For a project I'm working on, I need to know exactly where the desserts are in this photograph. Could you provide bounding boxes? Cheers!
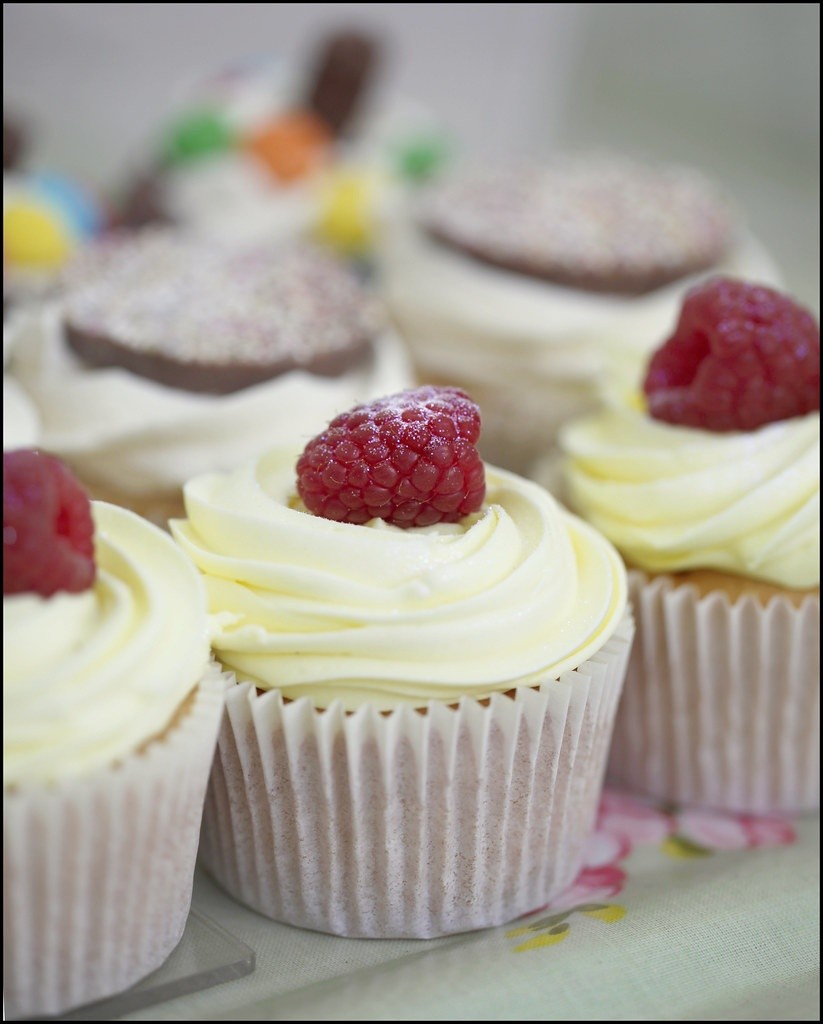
[2,30,821,1024]
